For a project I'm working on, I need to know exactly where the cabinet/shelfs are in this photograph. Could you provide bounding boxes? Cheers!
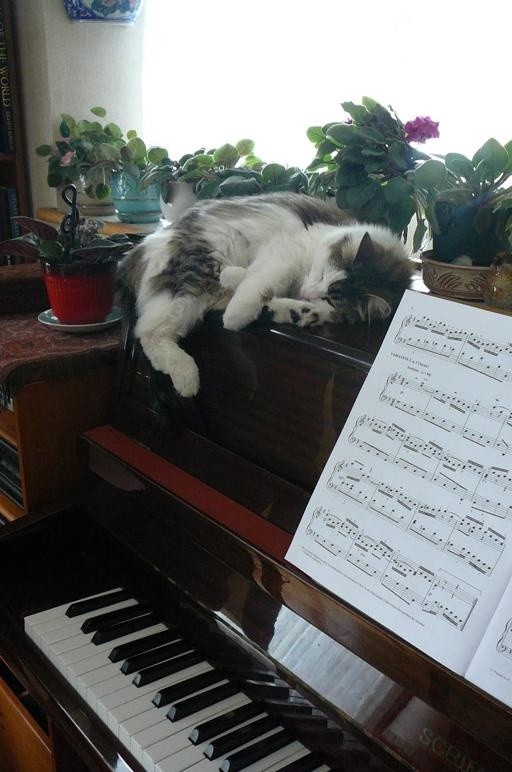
[0,261,119,526]
[0,0,33,265]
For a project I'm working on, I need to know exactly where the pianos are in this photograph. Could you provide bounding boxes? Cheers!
[0,280,512,772]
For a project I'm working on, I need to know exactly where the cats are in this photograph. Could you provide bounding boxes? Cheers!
[109,190,416,451]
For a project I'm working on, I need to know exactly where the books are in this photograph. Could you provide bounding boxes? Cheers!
[283,287,512,714]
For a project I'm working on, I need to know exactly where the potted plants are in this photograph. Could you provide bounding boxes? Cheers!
[0,96,512,336]
